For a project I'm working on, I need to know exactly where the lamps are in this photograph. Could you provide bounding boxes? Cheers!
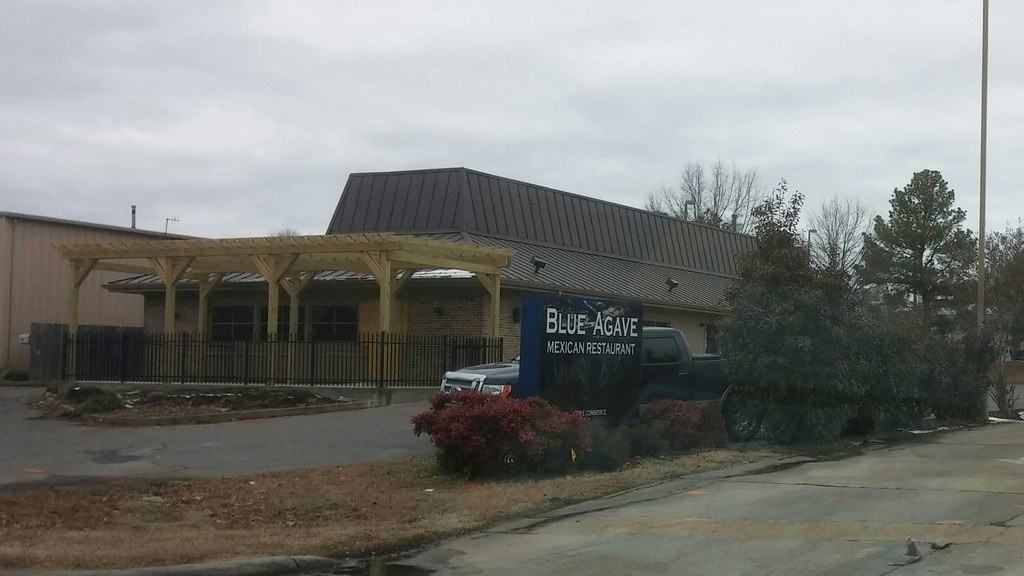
[531,255,546,274]
[174,312,180,318]
[665,277,679,292]
[433,301,440,313]
[699,323,705,327]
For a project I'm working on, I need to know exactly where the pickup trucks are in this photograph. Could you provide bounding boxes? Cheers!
[441,325,766,442]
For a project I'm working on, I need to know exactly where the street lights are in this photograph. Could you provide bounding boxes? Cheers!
[684,200,695,219]
[809,229,816,254]
